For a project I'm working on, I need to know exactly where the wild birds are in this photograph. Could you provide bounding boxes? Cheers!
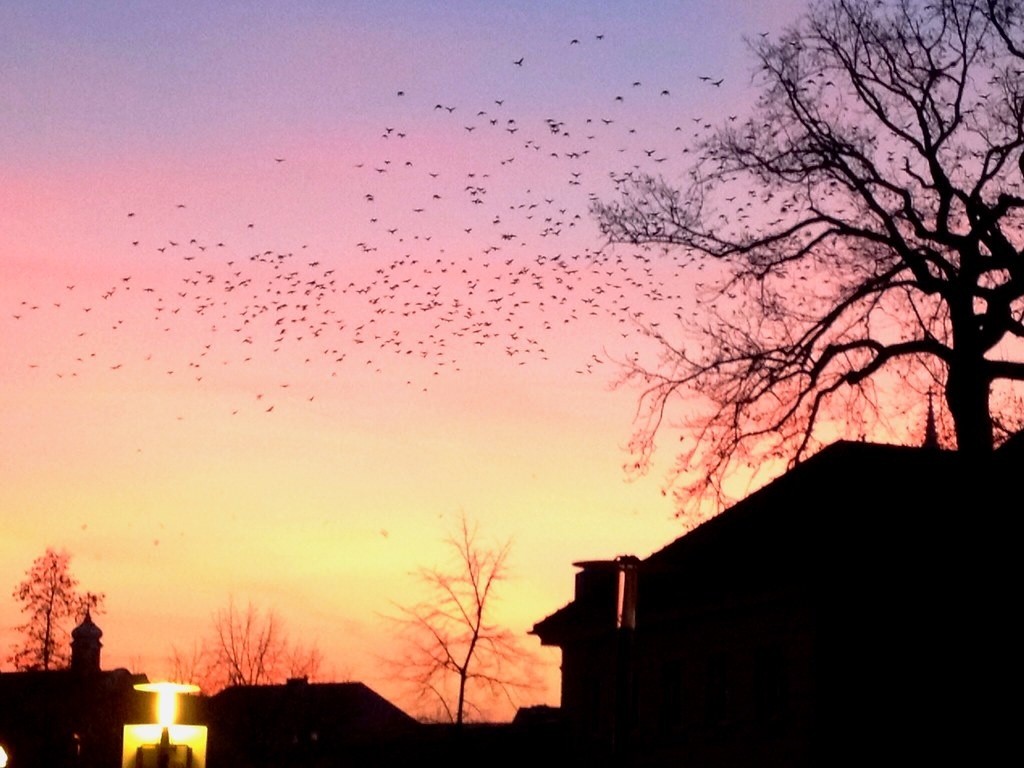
[11,4,1023,423]
